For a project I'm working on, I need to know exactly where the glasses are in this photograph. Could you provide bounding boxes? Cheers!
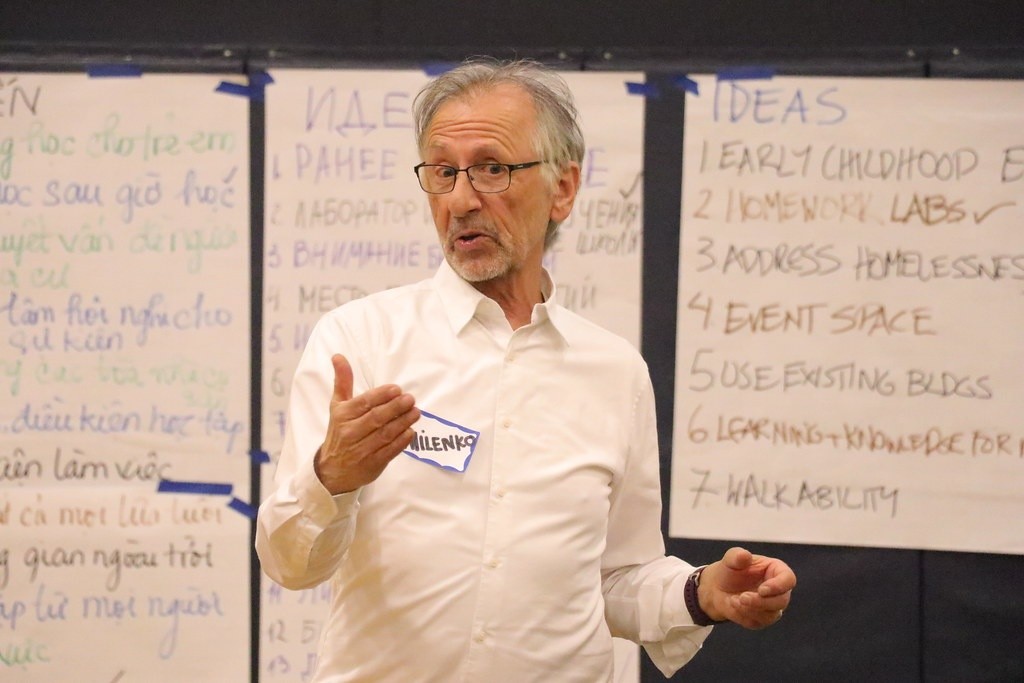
[414,160,548,194]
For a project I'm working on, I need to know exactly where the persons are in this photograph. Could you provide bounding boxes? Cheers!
[256,62,796,683]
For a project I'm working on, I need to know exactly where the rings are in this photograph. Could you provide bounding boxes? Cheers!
[779,609,783,620]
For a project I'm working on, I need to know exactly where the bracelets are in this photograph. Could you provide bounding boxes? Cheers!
[683,566,721,626]
